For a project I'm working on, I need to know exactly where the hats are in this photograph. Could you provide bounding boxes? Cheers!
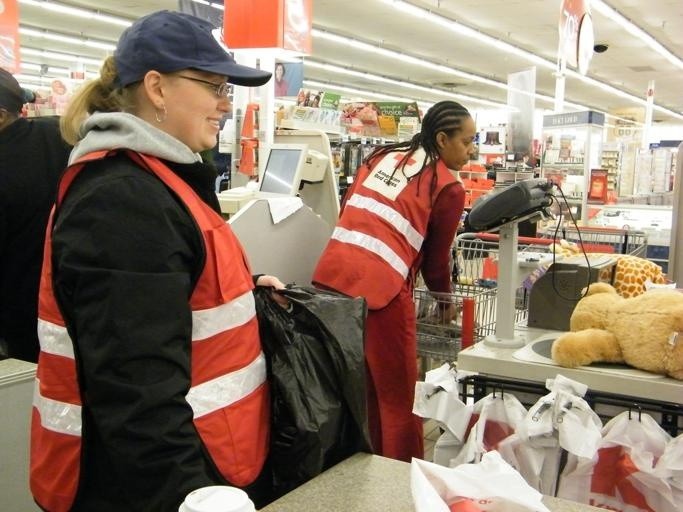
[114,10,272,88]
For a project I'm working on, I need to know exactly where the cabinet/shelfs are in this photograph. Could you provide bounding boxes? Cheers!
[447,110,683,275]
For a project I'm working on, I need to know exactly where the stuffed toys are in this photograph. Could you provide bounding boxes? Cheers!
[550,281,683,383]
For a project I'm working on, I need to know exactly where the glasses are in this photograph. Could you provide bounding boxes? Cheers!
[124,76,231,97]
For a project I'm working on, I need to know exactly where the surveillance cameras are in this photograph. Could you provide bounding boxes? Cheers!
[593,42,609,53]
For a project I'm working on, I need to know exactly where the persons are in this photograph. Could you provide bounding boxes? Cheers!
[27,8,289,511]
[0,66,72,364]
[310,99,477,464]
[275,63,288,96]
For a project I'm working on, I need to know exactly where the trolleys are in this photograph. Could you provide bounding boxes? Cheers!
[412,225,648,381]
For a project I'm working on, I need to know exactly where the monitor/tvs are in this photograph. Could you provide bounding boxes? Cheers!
[255,143,310,198]
